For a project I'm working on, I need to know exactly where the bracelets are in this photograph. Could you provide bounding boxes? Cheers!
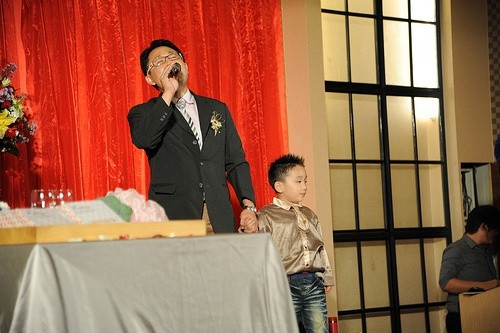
[244,206,257,212]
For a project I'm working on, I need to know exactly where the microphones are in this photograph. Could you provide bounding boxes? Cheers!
[171,63,181,74]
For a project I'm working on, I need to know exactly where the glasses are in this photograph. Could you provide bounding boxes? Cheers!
[147,53,183,78]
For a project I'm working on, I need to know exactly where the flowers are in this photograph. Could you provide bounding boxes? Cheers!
[211,111,225,136]
[0,62,36,157]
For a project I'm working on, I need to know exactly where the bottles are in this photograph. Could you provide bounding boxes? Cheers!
[328,317,338,333]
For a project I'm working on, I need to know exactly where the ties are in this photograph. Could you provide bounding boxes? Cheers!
[291,205,309,231]
[175,100,205,202]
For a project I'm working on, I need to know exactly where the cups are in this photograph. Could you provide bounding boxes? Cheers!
[31,189,73,208]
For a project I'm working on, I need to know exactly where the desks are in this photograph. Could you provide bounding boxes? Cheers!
[0,234,299,332]
[458,286,500,333]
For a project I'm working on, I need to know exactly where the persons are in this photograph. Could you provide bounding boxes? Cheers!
[439,205,500,333]
[127,39,257,234]
[246,154,334,333]
